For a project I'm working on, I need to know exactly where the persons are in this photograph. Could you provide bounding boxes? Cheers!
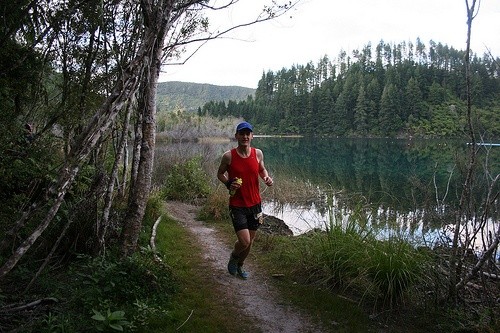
[216,122,274,281]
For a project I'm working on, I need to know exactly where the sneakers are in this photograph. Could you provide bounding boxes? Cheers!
[237,263,249,280]
[228,253,238,276]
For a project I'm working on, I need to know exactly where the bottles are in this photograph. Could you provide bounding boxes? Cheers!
[229,177,238,196]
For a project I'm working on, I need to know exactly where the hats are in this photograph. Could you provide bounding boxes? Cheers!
[236,122,253,132]
